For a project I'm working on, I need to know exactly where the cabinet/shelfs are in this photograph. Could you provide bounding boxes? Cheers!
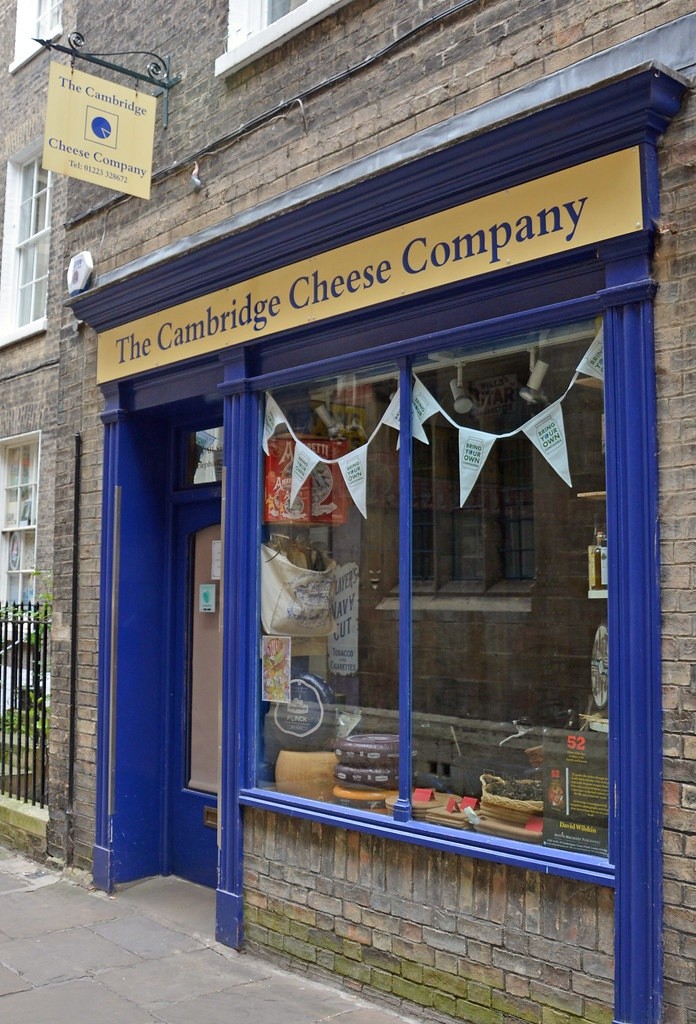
[263,399,366,525]
[574,377,610,733]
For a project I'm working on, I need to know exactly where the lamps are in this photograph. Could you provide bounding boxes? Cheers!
[519,347,550,403]
[449,362,473,413]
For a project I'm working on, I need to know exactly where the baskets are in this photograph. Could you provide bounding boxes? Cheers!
[480,773,544,824]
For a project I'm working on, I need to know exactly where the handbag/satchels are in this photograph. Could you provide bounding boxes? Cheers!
[261,542,337,637]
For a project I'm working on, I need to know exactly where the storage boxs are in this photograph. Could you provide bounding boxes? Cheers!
[264,400,365,524]
